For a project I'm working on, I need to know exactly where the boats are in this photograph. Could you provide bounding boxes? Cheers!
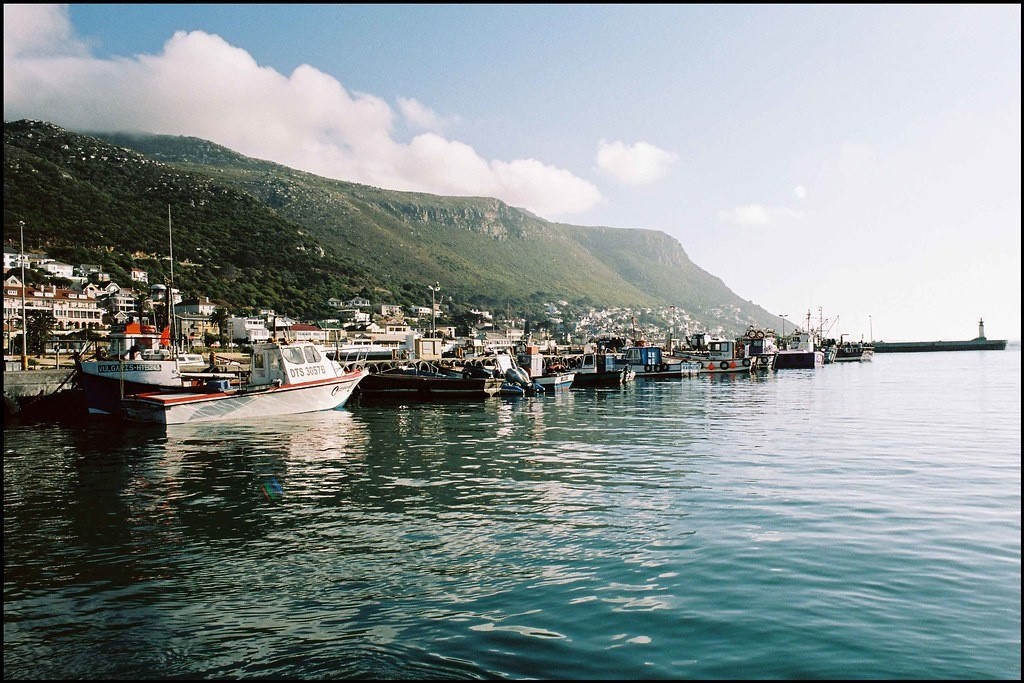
[355,307,838,403]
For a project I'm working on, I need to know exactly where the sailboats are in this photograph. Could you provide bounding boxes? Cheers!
[75,206,372,425]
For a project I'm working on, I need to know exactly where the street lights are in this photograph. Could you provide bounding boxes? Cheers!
[778,314,788,337]
[428,281,441,355]
[18,221,28,371]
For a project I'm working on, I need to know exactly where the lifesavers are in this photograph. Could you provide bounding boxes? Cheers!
[678,355,768,370]
[541,355,584,373]
[643,362,670,372]
[748,330,765,339]
[347,358,502,378]
